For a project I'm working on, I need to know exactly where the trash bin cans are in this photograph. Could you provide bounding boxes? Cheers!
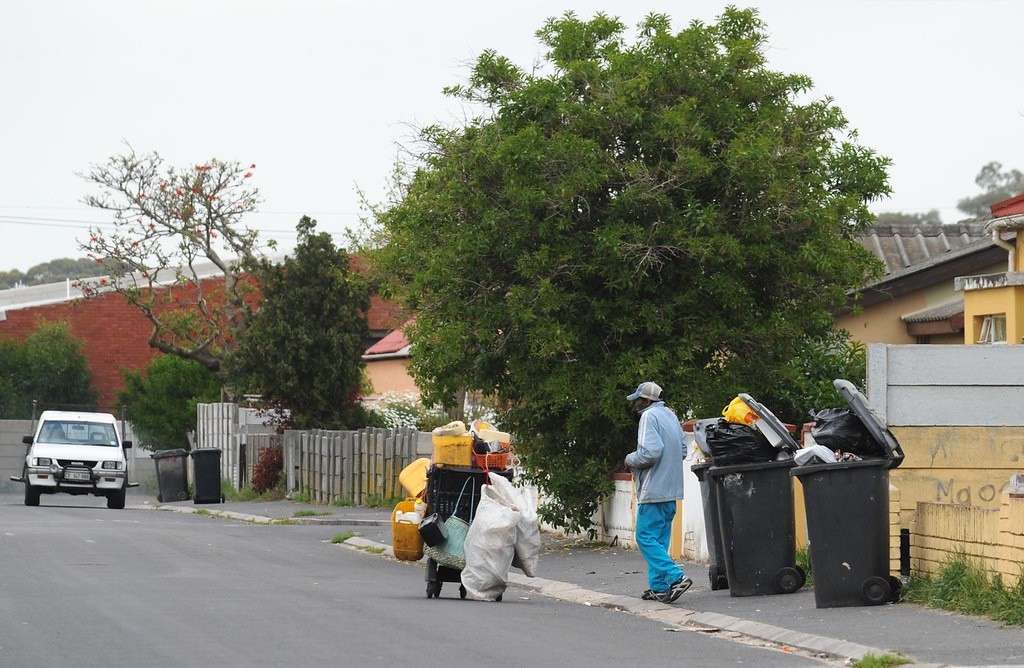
[788,378,905,610]
[150,448,190,502]
[691,392,807,600]
[189,447,226,504]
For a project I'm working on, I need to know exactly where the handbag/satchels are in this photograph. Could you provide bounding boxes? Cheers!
[423,475,474,570]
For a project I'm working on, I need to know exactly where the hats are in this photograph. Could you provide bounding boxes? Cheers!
[627,381,664,401]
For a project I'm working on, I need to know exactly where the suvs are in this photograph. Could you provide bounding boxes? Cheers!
[11,409,139,509]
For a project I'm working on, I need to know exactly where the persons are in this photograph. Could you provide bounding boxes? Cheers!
[51,423,62,438]
[624,382,693,602]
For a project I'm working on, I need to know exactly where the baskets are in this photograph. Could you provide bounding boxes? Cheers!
[472,440,510,470]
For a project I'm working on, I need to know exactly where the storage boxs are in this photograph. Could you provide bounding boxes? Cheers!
[429,432,473,467]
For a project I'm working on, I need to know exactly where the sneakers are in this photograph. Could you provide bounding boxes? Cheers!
[662,575,692,603]
[642,589,668,600]
